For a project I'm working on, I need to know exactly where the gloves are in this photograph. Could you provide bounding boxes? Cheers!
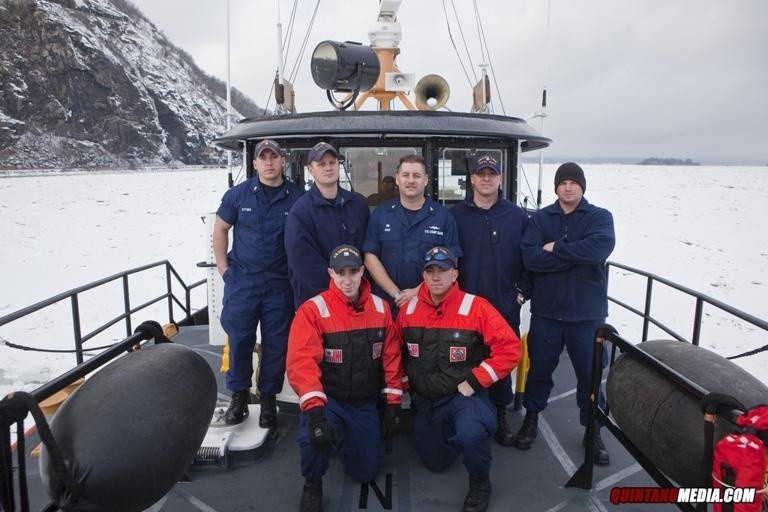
[308,411,338,449]
[379,405,400,430]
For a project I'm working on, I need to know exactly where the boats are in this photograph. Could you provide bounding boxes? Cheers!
[0,1,767,512]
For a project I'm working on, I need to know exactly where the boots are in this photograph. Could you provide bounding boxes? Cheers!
[300,479,322,512]
[225,391,248,424]
[496,407,513,447]
[259,392,276,427]
[583,427,609,466]
[463,475,492,512]
[516,411,537,450]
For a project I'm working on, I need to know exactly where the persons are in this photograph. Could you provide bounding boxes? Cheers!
[381,244,524,512]
[513,161,614,468]
[446,156,532,448]
[285,243,402,510]
[366,174,401,206]
[284,140,370,310]
[211,138,305,430]
[358,154,465,473]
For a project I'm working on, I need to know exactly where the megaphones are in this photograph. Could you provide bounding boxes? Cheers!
[384,72,416,91]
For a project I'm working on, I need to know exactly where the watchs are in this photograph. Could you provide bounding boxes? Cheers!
[516,295,525,305]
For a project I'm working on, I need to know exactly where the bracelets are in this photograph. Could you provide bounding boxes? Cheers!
[392,290,400,301]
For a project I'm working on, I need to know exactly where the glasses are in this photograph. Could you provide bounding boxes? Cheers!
[423,253,456,265]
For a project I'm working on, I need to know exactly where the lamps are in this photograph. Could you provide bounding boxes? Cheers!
[309,37,379,108]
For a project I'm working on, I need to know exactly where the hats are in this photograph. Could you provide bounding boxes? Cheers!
[555,163,586,194]
[329,244,362,273]
[256,140,281,160]
[306,142,338,165]
[422,247,457,272]
[471,156,500,175]
